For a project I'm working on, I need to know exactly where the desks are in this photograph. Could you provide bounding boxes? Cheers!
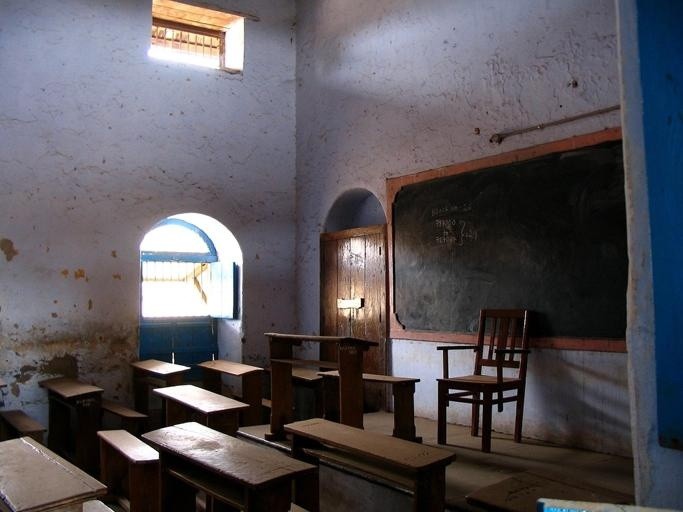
[0,332,636,511]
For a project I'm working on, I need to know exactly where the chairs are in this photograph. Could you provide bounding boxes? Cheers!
[436,308,533,453]
